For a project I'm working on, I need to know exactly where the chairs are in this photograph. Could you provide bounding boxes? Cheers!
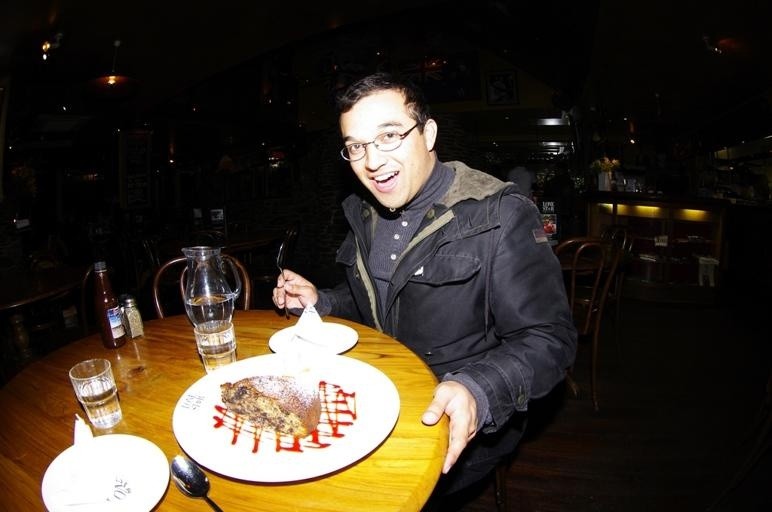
[1,204,637,412]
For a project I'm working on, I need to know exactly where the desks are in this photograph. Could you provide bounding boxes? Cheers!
[0,304,445,512]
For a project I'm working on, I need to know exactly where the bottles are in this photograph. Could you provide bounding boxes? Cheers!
[125,295,144,337]
[610,171,617,191]
[93,259,126,349]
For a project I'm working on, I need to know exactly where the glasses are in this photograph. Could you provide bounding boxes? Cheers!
[340,122,419,162]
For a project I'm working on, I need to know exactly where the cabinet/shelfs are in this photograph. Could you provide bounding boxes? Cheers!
[584,193,730,311]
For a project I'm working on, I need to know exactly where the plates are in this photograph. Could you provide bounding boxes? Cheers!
[268,320,359,355]
[170,353,400,487]
[42,435,171,512]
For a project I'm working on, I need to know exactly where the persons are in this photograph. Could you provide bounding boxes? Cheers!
[543,216,556,233]
[271,73,580,511]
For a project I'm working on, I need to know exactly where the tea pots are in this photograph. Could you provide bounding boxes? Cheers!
[177,245,243,327]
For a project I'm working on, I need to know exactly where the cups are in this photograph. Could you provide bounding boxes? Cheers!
[617,185,624,192]
[191,320,237,371]
[69,358,124,430]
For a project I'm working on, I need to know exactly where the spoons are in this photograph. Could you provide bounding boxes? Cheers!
[276,241,291,319]
[171,454,221,511]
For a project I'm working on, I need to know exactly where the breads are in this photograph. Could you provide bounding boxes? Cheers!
[219,375,322,438]
